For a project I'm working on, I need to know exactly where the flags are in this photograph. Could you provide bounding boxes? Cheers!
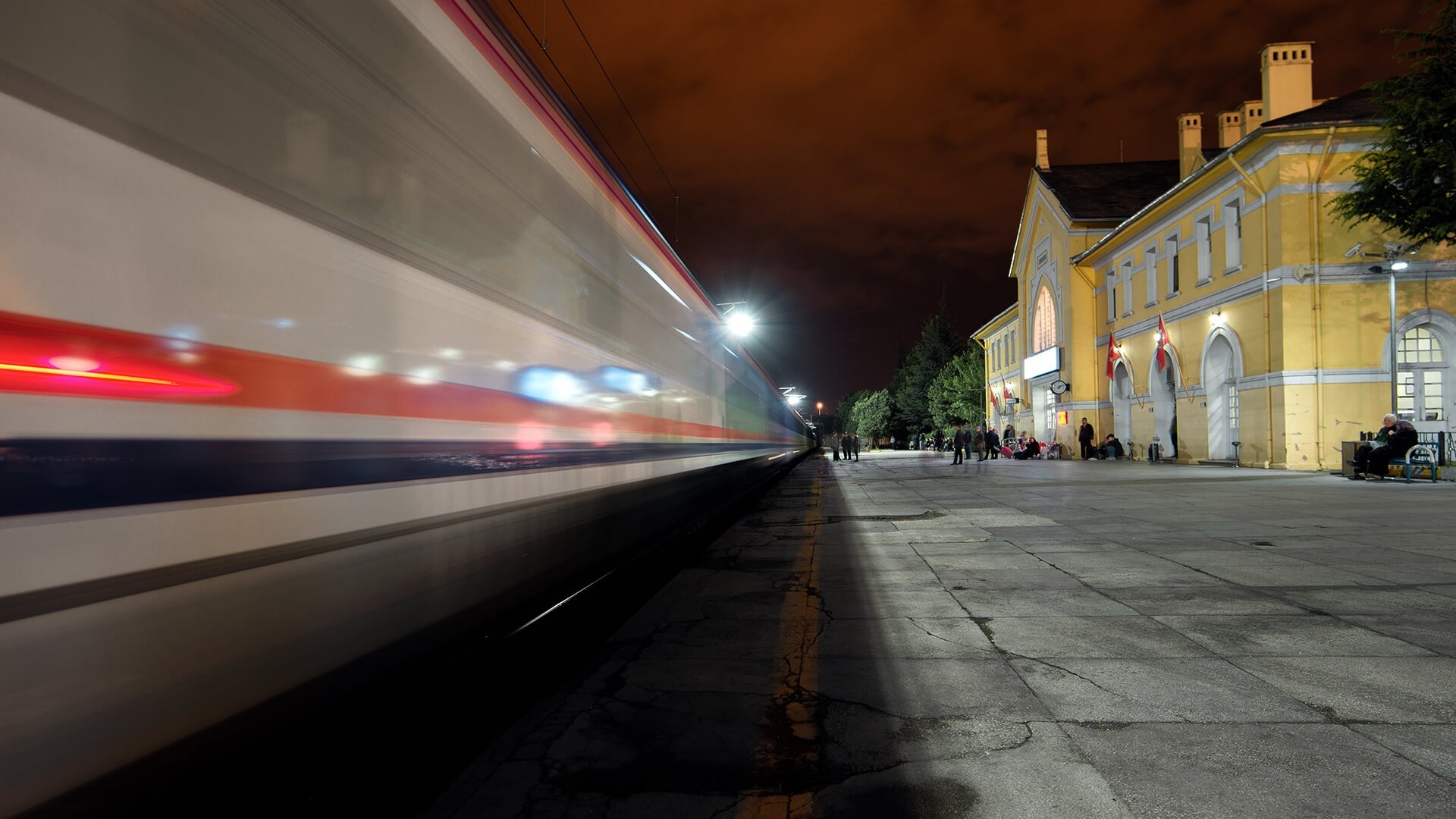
[1156,314,1172,373]
[1105,332,1124,380]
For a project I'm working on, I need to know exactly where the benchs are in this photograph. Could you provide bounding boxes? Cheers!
[1389,441,1440,483]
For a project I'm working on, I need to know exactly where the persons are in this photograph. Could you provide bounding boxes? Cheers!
[1078,417,1094,461]
[983,426,995,460]
[1104,433,1123,461]
[975,426,984,461]
[1017,438,1027,452]
[950,424,963,465]
[993,427,1001,460]
[1346,413,1398,479]
[1087,437,1109,461]
[919,430,952,452]
[1003,424,1016,450]
[853,433,859,462]
[841,432,847,460]
[963,428,972,460]
[890,436,895,450]
[831,431,841,462]
[846,431,852,460]
[1366,420,1418,479]
[1026,437,1041,459]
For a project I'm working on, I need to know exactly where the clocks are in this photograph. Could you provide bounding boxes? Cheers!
[1050,380,1070,394]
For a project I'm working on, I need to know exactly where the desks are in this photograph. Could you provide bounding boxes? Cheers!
[1341,441,1368,480]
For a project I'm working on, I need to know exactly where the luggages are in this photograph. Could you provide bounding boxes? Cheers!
[1000,446,1013,460]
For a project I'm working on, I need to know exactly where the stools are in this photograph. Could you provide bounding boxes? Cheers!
[1232,442,1241,467]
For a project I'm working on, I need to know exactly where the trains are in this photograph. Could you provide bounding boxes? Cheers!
[0,0,817,819]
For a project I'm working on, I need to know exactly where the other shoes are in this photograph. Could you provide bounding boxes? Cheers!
[1087,458,1098,461]
[1359,472,1382,480]
[1346,460,1361,469]
[976,459,984,461]
[1105,456,1117,460]
[949,462,963,465]
[1348,474,1366,480]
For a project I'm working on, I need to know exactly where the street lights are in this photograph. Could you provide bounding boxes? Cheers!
[816,402,824,446]
[1388,260,1408,422]
